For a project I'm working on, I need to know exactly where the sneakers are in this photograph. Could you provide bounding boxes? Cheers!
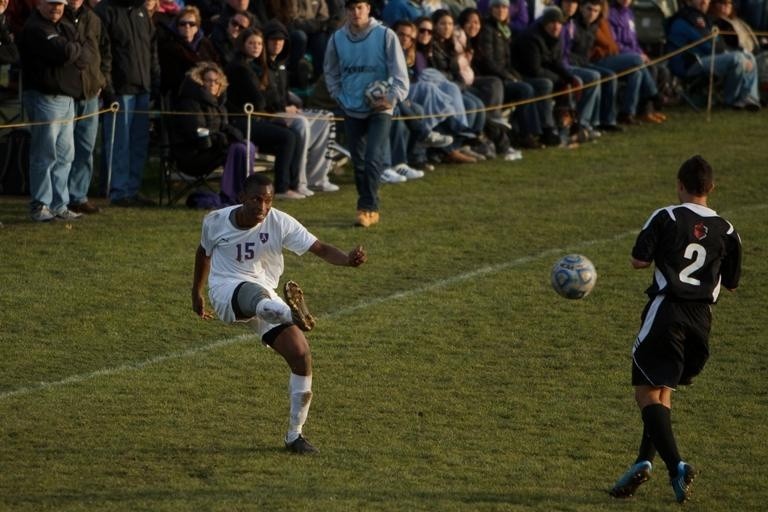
[32,202,56,221]
[391,162,424,180]
[283,279,316,332]
[670,460,697,504]
[70,195,101,215]
[378,169,408,184]
[726,98,761,111]
[356,207,380,227]
[54,208,86,221]
[274,177,340,199]
[608,462,651,499]
[284,431,320,456]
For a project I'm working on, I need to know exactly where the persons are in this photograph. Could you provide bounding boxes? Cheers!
[15,1,100,221]
[321,0,411,228]
[608,153,745,504]
[191,171,370,458]
[2,0,768,214]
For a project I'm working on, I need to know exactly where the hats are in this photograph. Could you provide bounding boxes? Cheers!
[542,4,567,30]
[419,27,433,36]
[488,0,510,9]
[268,31,288,41]
[44,0,67,5]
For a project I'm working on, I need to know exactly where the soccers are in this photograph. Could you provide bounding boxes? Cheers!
[551,254,597,300]
[364,80,390,109]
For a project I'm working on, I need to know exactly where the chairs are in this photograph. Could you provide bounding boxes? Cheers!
[155,113,231,210]
[659,37,727,115]
[625,1,671,72]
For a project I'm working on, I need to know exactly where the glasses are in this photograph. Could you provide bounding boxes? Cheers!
[178,20,198,28]
[231,17,248,31]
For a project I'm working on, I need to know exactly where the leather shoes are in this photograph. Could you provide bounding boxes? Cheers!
[110,193,155,208]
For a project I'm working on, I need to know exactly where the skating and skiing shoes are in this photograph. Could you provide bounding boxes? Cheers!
[422,111,667,172]
